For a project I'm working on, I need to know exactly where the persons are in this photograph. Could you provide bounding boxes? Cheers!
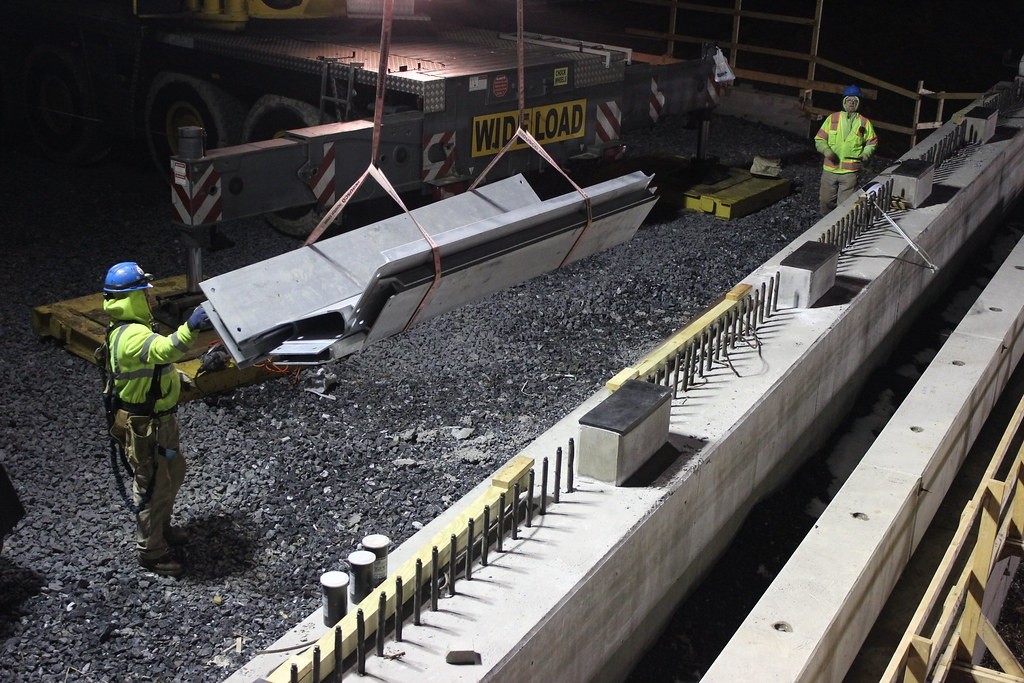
[813,85,878,218]
[102,262,214,574]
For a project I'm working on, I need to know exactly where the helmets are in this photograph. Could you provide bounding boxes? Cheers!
[842,85,862,99]
[103,261,153,292]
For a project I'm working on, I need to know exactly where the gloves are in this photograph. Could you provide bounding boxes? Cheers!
[175,369,196,392]
[859,153,870,162]
[188,305,211,331]
[824,149,837,162]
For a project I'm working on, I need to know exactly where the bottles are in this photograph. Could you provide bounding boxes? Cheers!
[362,533,389,586]
[349,550,376,605]
[320,570,350,627]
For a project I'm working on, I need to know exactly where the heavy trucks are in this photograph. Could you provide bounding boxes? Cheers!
[0,0,736,334]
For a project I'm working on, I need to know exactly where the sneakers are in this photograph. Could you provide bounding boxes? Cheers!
[162,525,189,546]
[137,550,183,575]
